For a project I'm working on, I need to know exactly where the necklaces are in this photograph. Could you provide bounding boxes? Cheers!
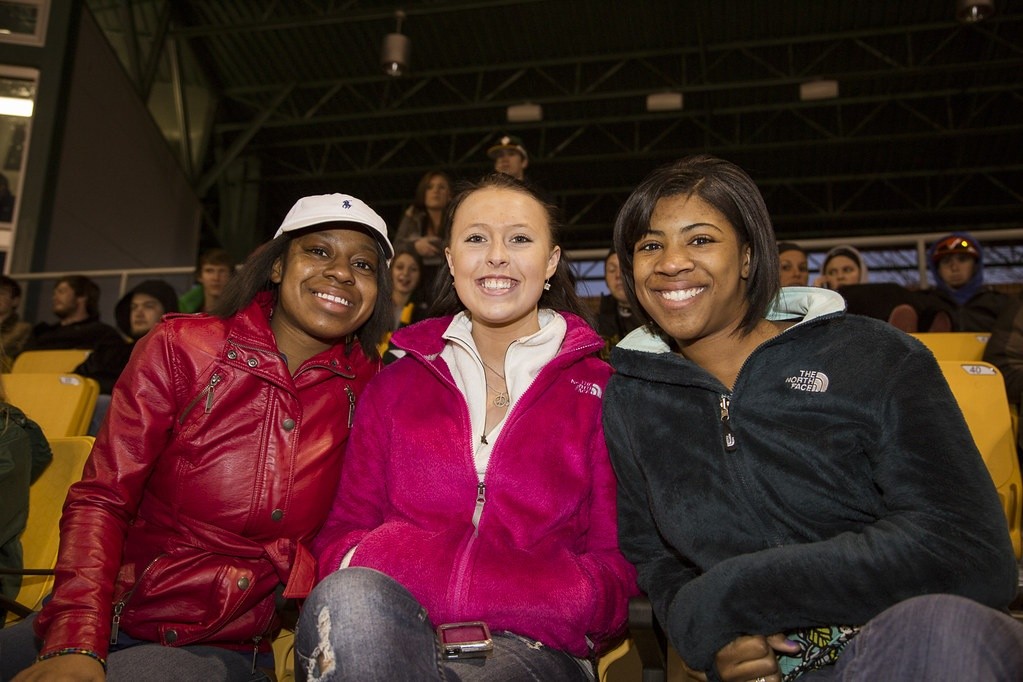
[482,361,509,380]
[486,383,510,407]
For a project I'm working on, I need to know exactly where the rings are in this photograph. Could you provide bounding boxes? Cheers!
[756,676,767,682]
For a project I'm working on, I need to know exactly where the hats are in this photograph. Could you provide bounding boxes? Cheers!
[273,193,394,268]
[488,135,528,161]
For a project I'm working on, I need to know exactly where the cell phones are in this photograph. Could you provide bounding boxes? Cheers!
[435,621,494,660]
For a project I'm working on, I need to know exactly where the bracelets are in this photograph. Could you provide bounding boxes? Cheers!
[37,647,108,676]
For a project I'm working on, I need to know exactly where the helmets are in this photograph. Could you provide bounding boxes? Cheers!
[930,240,980,267]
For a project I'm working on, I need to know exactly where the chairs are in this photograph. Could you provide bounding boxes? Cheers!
[1,333,1023,682]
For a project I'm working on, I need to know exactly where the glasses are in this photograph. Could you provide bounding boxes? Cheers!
[936,242,969,253]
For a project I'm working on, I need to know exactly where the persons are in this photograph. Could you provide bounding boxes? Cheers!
[600,154,1022,682]
[1,370,53,629]
[0,191,403,682]
[1,129,1022,421]
[292,173,642,681]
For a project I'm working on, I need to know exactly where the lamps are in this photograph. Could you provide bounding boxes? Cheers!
[954,0,995,24]
[379,10,410,77]
[800,79,839,101]
[645,90,685,111]
[508,101,542,122]
